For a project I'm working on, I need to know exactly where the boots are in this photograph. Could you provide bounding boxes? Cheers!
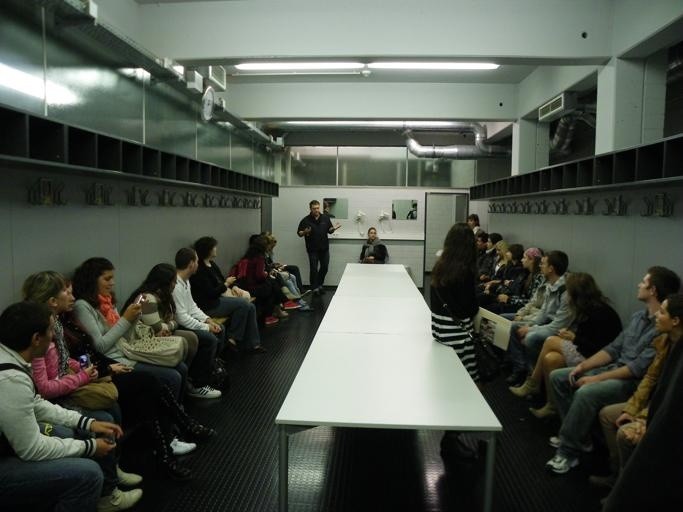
[273,304,288,317]
[528,394,558,419]
[509,376,541,401]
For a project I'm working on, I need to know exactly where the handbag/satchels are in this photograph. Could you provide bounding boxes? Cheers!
[264,271,283,291]
[208,357,227,384]
[221,287,250,302]
[118,336,188,366]
[473,333,503,374]
[59,368,118,409]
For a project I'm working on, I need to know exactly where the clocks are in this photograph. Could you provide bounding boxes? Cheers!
[200,84,215,123]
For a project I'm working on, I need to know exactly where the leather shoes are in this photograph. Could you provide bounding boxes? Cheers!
[116,463,143,485]
[97,488,143,511]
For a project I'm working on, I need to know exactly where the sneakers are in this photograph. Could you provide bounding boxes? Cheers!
[184,425,216,442]
[548,437,593,452]
[590,472,615,488]
[264,317,279,324]
[313,288,320,296]
[294,300,309,311]
[545,455,579,473]
[441,434,474,458]
[283,300,302,309]
[168,465,192,479]
[301,288,311,298]
[225,337,239,352]
[246,346,267,353]
[169,439,196,455]
[319,287,326,294]
[191,385,222,398]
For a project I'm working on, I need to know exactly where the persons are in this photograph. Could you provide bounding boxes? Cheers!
[406,203,417,220]
[430,214,683,512]
[322,201,335,219]
[297,199,341,294]
[0,232,310,511]
[359,227,389,264]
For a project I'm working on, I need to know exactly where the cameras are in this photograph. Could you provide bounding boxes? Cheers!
[137,294,145,305]
[79,354,91,367]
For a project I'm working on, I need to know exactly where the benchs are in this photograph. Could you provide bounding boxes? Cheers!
[173,293,256,330]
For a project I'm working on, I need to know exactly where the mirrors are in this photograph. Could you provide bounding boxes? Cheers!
[391,199,418,221]
[322,198,349,219]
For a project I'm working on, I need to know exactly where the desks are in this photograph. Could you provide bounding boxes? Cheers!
[273,333,505,512]
[334,262,422,296]
[316,295,433,334]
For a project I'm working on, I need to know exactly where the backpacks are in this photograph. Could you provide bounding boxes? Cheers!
[229,256,248,287]
[407,210,416,219]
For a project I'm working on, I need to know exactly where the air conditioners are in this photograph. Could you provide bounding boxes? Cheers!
[536,91,577,123]
[187,64,227,93]
